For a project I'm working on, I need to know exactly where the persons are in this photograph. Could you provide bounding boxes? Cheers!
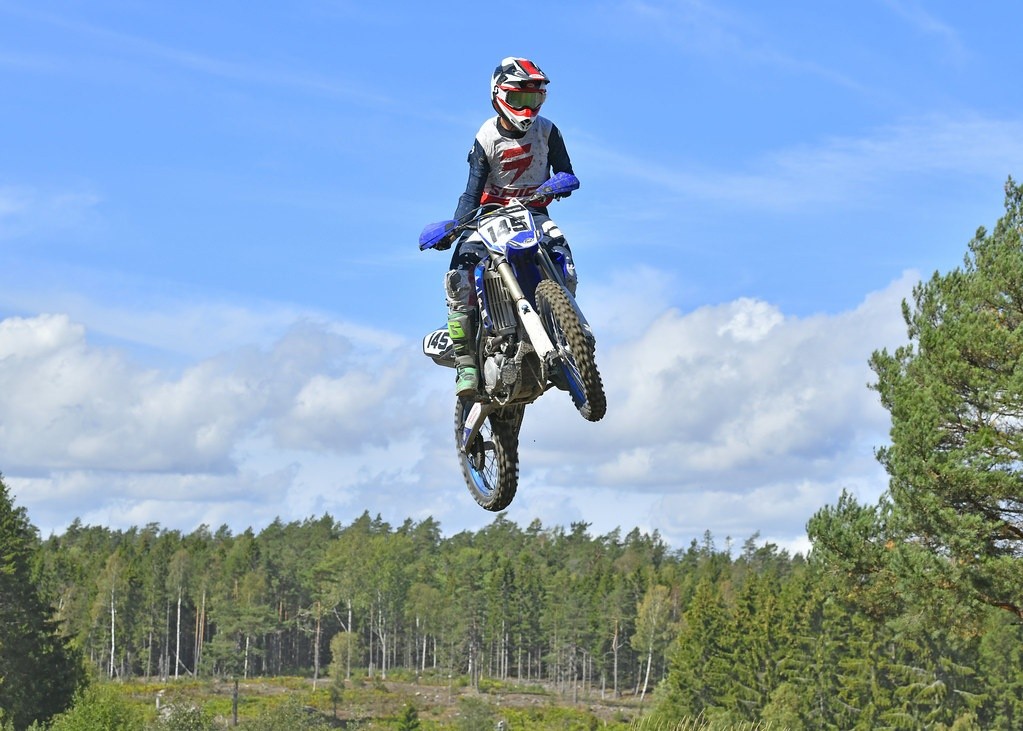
[444,57,580,398]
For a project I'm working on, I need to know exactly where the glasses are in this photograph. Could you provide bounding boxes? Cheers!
[496,87,547,111]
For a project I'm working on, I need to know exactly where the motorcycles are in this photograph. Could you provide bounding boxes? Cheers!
[418,172,604,514]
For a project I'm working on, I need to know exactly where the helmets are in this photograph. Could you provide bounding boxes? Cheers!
[491,57,550,132]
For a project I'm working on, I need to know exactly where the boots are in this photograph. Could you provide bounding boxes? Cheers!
[447,309,480,396]
